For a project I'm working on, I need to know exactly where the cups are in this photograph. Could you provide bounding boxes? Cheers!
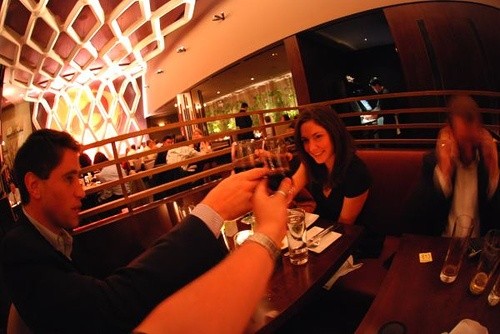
[178,201,196,219]
[230,136,294,196]
[440,214,476,284]
[487,271,500,306]
[286,208,308,264]
[225,220,237,237]
[469,229,500,296]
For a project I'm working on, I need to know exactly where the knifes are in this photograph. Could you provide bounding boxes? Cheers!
[308,224,339,247]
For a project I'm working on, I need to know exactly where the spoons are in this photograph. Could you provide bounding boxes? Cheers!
[309,226,333,242]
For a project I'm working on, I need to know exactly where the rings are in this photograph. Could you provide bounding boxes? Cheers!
[277,189,287,197]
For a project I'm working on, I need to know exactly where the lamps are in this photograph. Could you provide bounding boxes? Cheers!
[212,12,225,21]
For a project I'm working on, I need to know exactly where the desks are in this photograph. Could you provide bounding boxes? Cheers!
[219,201,365,334]
[355,235,500,334]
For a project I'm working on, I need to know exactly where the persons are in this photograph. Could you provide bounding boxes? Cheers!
[288,108,370,262]
[131,176,292,334]
[235,102,255,138]
[94,152,132,209]
[264,116,274,143]
[362,77,394,124]
[8,182,22,204]
[0,128,270,334]
[125,128,214,195]
[402,95,500,238]
[281,114,294,128]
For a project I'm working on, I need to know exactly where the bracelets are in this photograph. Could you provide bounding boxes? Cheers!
[247,233,280,262]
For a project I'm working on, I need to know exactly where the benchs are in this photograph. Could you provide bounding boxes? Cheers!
[313,150,434,295]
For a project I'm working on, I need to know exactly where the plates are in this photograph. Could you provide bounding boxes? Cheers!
[233,210,289,250]
[306,212,319,228]
